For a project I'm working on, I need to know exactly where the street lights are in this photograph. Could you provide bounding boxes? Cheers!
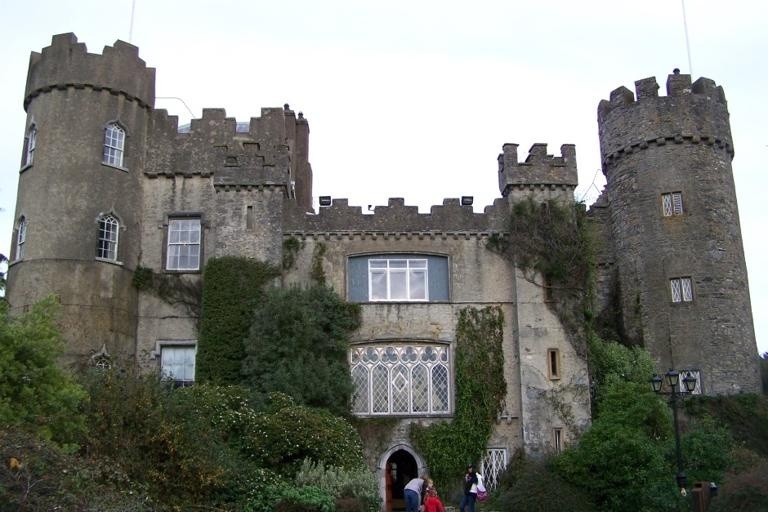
[644,366,700,492]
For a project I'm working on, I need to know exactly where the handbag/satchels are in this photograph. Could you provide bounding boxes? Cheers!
[476,485,487,500]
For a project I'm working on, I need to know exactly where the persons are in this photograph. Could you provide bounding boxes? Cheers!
[404,474,446,512]
[459,464,486,512]
